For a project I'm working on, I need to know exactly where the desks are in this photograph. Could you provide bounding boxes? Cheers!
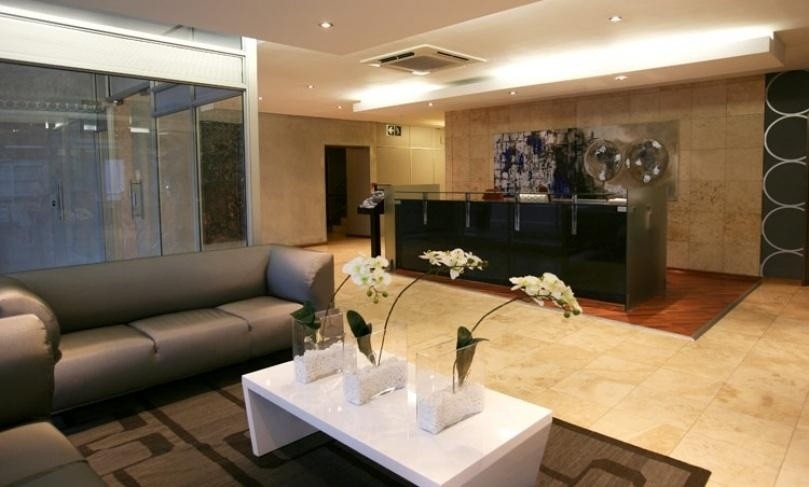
[383,182,668,313]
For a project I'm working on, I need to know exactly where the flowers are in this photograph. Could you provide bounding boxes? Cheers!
[383,248,488,313]
[325,255,392,314]
[458,272,582,344]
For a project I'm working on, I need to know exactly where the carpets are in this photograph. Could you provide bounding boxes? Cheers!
[43,346,713,486]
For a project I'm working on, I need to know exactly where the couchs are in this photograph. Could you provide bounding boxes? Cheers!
[2,242,335,415]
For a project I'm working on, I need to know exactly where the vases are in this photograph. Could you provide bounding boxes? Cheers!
[290,306,488,435]
